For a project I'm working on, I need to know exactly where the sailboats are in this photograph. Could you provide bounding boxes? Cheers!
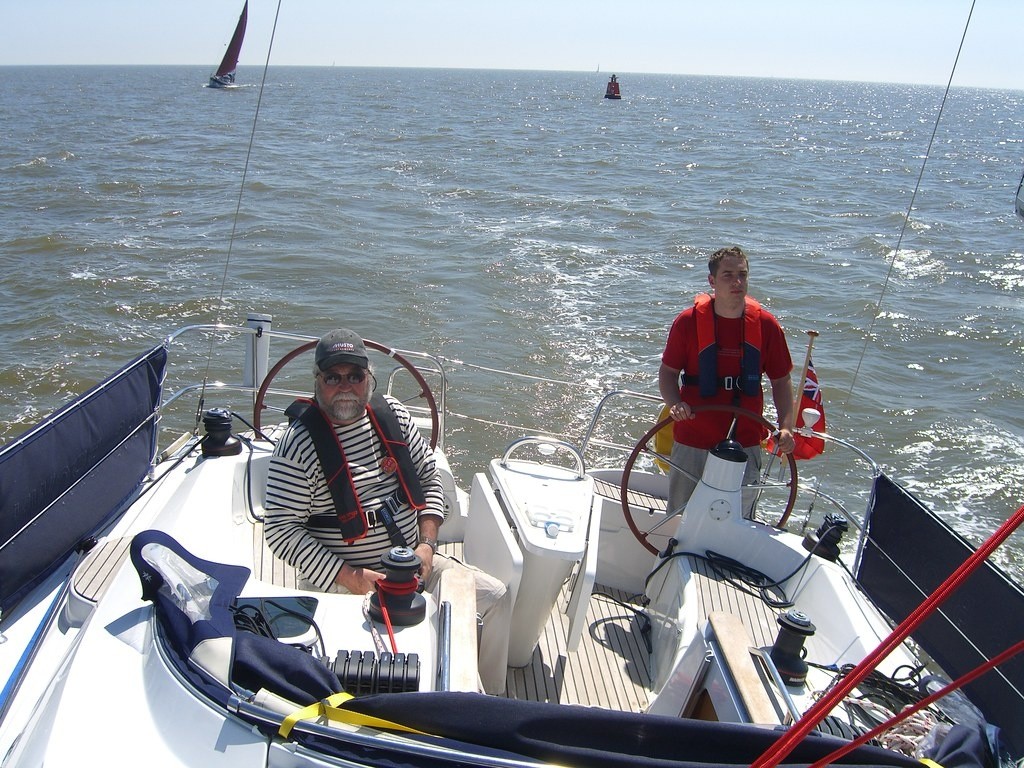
[207,0,249,89]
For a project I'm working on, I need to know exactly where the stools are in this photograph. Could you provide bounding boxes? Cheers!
[592,476,679,516]
[253,521,463,593]
[679,555,784,649]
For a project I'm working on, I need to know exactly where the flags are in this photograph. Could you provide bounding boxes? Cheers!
[766,349,826,460]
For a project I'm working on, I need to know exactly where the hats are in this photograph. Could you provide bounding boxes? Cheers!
[314,327,369,371]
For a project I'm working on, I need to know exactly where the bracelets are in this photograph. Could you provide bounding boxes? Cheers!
[419,537,438,555]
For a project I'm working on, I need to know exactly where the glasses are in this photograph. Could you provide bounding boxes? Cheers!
[315,368,368,387]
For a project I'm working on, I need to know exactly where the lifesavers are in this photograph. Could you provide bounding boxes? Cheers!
[654,401,674,476]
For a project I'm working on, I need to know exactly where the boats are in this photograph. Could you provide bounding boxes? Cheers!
[604,73,621,99]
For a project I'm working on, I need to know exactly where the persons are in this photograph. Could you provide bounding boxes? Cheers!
[263,328,512,701]
[658,245,796,520]
